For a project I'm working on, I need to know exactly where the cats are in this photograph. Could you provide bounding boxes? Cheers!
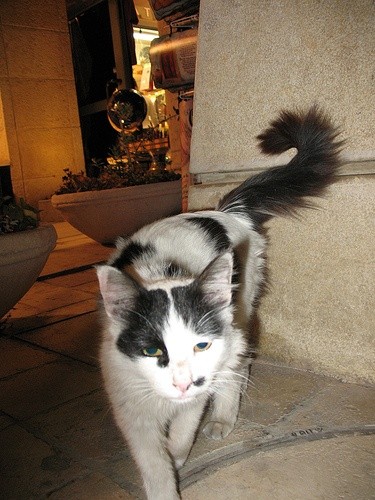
[92,103,354,500]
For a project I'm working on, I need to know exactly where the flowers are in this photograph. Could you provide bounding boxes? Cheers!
[56,164,181,191]
[0,195,41,234]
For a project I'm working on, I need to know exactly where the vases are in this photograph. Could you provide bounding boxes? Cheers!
[51,180,182,243]
[0,224,58,319]
